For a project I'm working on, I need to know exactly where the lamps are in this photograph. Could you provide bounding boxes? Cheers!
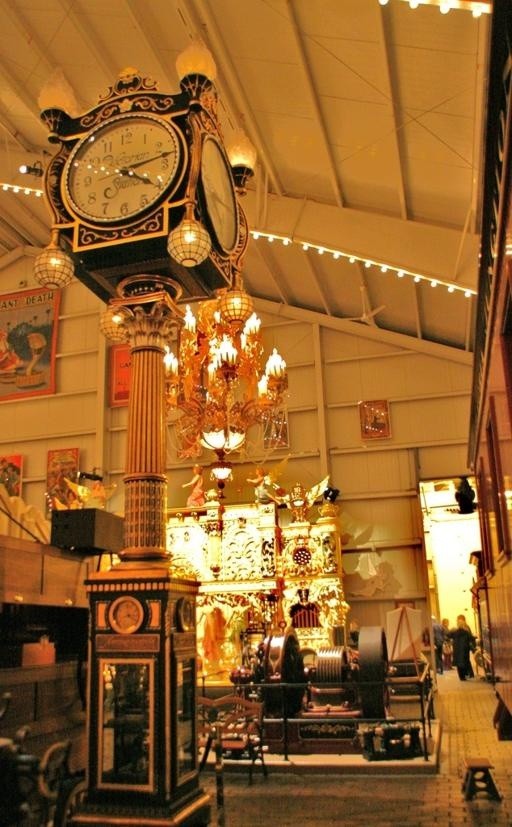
[160,290,290,486]
[25,35,256,200]
[28,205,255,346]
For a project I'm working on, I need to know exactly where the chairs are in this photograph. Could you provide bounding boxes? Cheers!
[195,693,270,787]
[1,725,76,826]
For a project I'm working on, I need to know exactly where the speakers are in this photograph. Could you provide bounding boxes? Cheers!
[50,508,125,556]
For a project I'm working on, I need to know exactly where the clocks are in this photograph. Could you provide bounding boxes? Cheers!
[106,594,149,637]
[53,111,244,255]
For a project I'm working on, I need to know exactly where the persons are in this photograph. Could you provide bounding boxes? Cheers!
[180,463,207,506]
[446,619,477,680]
[195,593,227,664]
[440,617,455,671]
[224,596,251,663]
[456,614,474,676]
[245,466,281,505]
[47,466,80,509]
[430,615,445,674]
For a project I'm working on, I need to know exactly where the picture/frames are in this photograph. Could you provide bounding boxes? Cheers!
[106,344,132,408]
[0,449,81,521]
[358,400,392,441]
[0,289,59,407]
[259,409,288,451]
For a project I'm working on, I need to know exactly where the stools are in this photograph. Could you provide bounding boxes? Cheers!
[463,756,502,798]
